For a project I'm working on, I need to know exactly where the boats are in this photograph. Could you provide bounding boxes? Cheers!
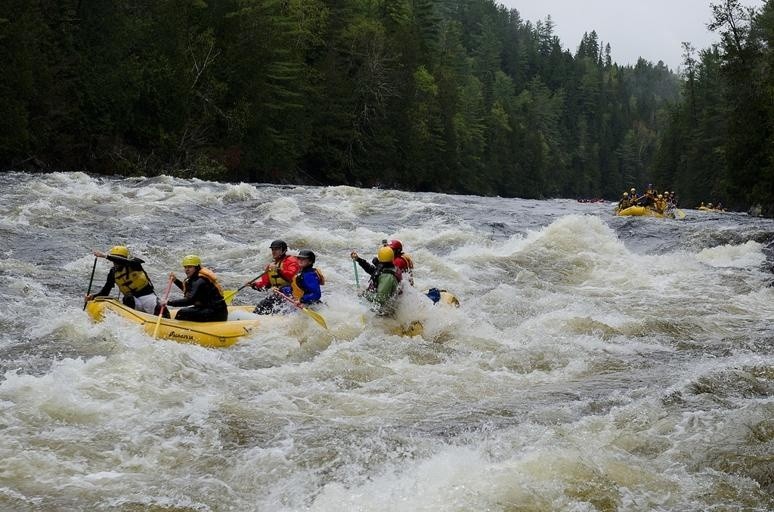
[614,207,726,219]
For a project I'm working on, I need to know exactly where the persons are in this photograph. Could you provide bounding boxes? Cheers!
[244,240,299,290]
[84,245,170,319]
[384,240,414,285]
[253,250,321,314]
[619,184,678,213]
[351,246,399,313]
[698,200,725,211]
[578,198,604,202]
[159,254,227,321]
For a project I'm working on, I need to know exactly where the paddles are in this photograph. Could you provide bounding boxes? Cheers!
[270,288,328,328]
[222,267,270,305]
[352,259,369,327]
[670,201,687,219]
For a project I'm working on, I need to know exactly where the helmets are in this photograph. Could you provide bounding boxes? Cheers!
[658,194,663,199]
[631,188,636,192]
[623,192,628,196]
[670,191,675,198]
[269,240,287,253]
[110,246,129,258]
[648,184,653,188]
[297,249,315,263]
[183,255,201,268]
[378,246,394,263]
[387,239,402,250]
[663,191,669,198]
